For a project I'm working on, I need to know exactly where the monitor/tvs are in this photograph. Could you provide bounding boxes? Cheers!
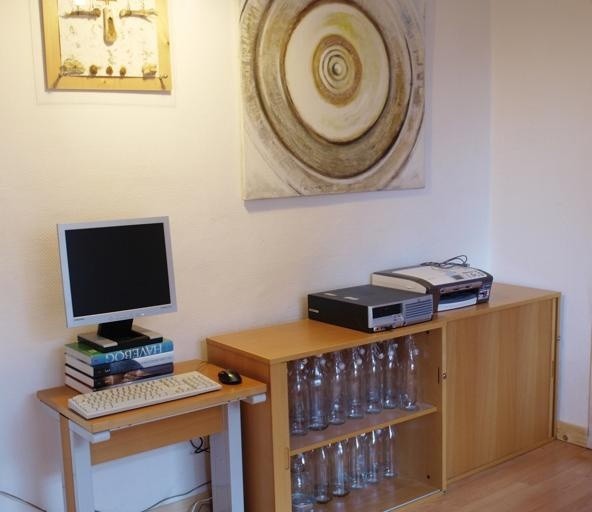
[56,216,178,353]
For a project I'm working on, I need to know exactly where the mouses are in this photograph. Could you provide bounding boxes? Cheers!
[218,370,241,384]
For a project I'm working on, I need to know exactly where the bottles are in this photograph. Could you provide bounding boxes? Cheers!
[290,334,418,436]
[291,426,396,512]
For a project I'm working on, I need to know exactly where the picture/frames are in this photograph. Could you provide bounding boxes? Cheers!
[39,1,172,93]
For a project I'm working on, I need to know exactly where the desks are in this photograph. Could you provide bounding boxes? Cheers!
[36,359,266,512]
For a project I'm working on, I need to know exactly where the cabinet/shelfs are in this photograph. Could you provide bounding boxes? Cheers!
[207,318,445,512]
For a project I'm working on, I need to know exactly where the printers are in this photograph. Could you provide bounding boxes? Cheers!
[371,262,494,313]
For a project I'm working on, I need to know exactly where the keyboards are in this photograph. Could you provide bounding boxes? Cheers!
[67,370,222,420]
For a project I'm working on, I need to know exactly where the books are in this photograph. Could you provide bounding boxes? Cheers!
[63,338,175,395]
